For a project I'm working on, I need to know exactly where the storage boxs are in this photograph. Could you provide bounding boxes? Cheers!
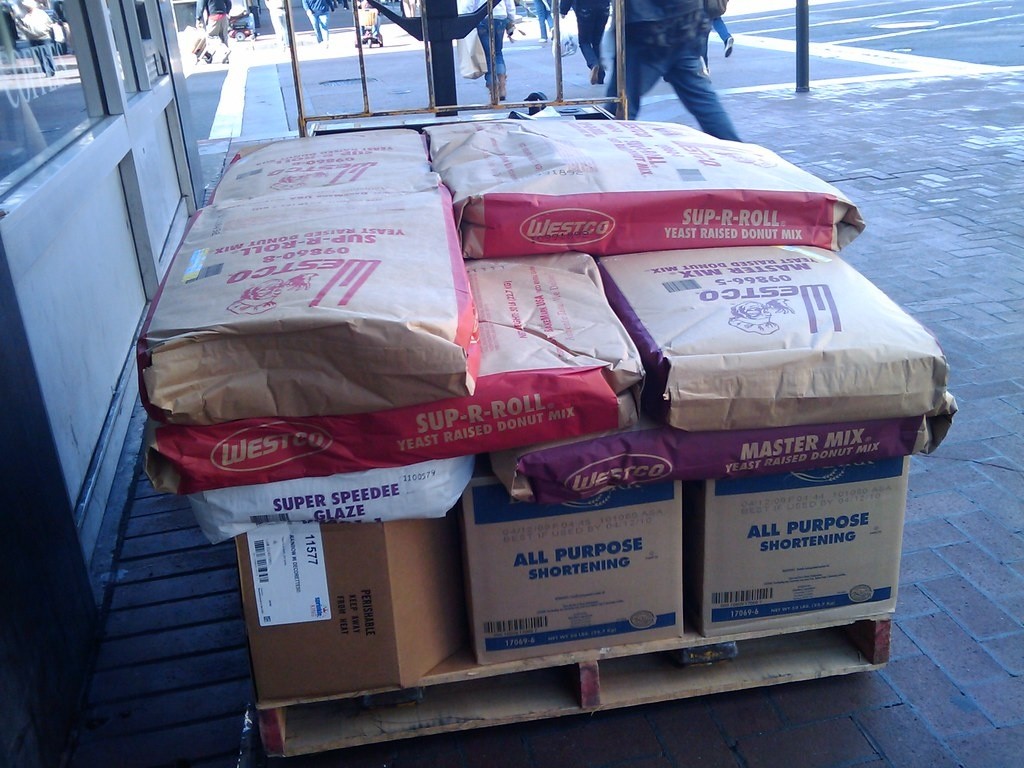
[460,459,683,666]
[235,509,469,702]
[683,454,912,639]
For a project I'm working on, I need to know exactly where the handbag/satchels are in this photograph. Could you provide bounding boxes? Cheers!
[552,17,577,56]
[457,28,488,79]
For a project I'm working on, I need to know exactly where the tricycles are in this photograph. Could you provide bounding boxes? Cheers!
[354,16,383,49]
[225,13,257,42]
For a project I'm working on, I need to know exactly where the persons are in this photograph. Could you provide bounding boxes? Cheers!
[534,0,554,42]
[244,0,261,35]
[357,0,381,44]
[457,0,516,101]
[604,0,741,142]
[196,0,232,64]
[700,17,734,75]
[560,0,610,85]
[17,0,56,77]
[302,0,334,49]
[265,0,289,47]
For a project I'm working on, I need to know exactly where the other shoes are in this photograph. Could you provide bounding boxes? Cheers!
[551,27,554,39]
[590,65,605,85]
[725,36,734,57]
[538,39,547,42]
[700,55,711,76]
